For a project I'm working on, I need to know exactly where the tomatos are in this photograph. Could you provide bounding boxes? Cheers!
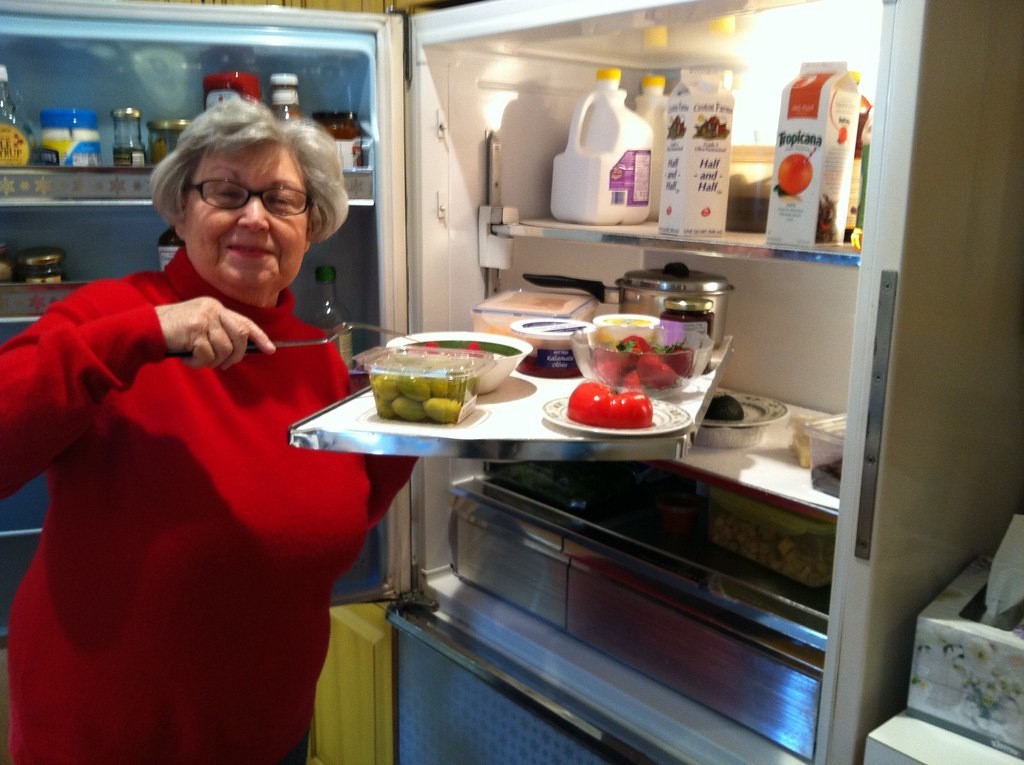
[567,383,653,429]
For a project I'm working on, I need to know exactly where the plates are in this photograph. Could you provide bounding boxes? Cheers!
[541,394,692,436]
[699,394,788,429]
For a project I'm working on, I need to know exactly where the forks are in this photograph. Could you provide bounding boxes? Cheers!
[166,321,419,358]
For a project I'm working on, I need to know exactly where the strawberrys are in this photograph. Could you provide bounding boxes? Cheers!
[592,336,694,390]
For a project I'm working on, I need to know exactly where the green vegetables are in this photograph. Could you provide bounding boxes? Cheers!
[501,457,635,503]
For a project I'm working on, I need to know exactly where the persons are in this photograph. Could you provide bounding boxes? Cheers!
[0,100,422,765]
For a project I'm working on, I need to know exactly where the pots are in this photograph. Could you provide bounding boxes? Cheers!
[522,262,739,347]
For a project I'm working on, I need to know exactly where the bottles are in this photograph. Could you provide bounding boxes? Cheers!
[311,265,352,370]
[112,107,145,168]
[843,71,872,244]
[551,67,653,227]
[270,73,301,122]
[634,74,668,220]
[1,64,38,168]
[851,105,872,249]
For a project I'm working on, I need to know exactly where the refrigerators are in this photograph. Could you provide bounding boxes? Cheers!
[0,1,1024,765]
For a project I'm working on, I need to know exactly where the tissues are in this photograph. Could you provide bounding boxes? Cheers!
[905,514,1024,757]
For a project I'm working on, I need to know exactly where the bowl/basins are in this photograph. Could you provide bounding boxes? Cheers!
[571,324,714,399]
[387,331,534,396]
[351,345,495,426]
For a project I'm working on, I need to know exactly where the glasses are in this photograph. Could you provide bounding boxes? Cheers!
[183,178,314,216]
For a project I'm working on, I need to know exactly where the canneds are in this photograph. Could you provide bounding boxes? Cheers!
[38,108,102,168]
[659,296,714,375]
[311,111,361,172]
[111,107,146,167]
[0,240,68,284]
[146,118,192,165]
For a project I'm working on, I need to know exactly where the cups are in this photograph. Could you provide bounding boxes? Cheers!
[655,492,699,534]
